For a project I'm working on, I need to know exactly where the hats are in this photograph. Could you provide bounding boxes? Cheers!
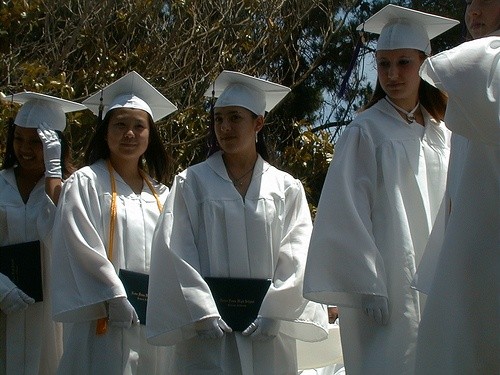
[204,71,291,151]
[82,71,177,161]
[356,5,461,56]
[2,91,87,168]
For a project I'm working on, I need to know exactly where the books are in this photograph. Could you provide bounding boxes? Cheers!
[110,268,149,326]
[0,240,45,304]
[198,275,271,331]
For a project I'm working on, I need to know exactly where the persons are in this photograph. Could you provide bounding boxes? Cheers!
[409,0,500,375]
[294,302,346,375]
[302,5,460,375]
[0,91,89,375]
[146,70,344,375]
[51,71,177,375]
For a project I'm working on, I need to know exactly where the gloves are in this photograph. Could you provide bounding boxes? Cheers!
[362,294,389,328]
[197,316,232,339]
[241,315,281,343]
[36,121,62,180]
[108,296,140,332]
[0,287,36,316]
